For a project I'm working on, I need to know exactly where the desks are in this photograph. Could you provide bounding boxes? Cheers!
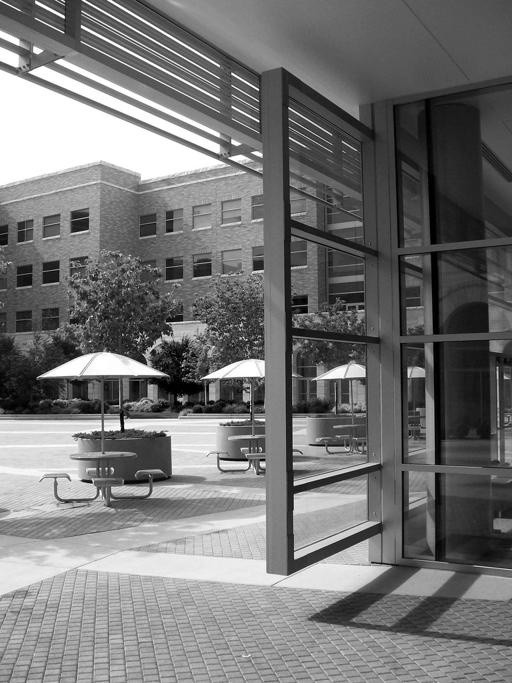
[408,415,426,440]
[228,435,265,470]
[70,452,138,499]
[333,424,365,453]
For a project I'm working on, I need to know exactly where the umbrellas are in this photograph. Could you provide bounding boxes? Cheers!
[199,358,306,451]
[35,350,171,477]
[309,358,368,435]
[403,364,428,415]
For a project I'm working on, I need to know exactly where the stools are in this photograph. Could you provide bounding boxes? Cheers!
[505,412,512,427]
[39,468,168,507]
[206,450,303,474]
[316,434,366,455]
[409,426,420,440]
